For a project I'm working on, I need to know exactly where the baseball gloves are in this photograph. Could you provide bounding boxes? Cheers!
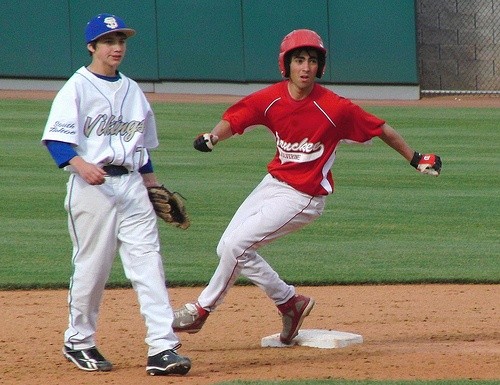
[146,185,192,230]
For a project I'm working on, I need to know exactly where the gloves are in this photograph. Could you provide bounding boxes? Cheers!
[193,133,218,153]
[410,151,442,178]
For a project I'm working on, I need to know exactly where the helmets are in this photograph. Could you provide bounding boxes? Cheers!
[278,29,326,79]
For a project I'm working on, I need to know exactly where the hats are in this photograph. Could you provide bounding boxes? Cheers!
[84,13,135,43]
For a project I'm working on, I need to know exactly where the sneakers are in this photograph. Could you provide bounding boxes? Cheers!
[62,345,113,372]
[146,343,192,376]
[276,295,315,345]
[171,301,210,335]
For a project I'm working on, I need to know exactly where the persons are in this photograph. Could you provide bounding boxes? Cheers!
[42,14,192,376]
[171,29,443,345]
